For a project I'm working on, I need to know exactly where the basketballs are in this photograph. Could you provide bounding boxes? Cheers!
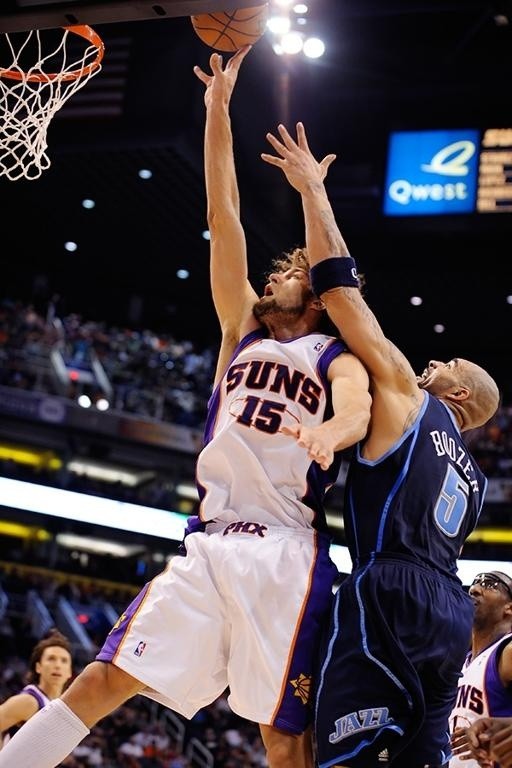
[191,0,269,51]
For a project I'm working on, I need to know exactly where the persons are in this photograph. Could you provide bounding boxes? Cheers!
[1,44,511,768]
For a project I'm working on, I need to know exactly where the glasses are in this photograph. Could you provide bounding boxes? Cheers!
[472,572,512,599]
